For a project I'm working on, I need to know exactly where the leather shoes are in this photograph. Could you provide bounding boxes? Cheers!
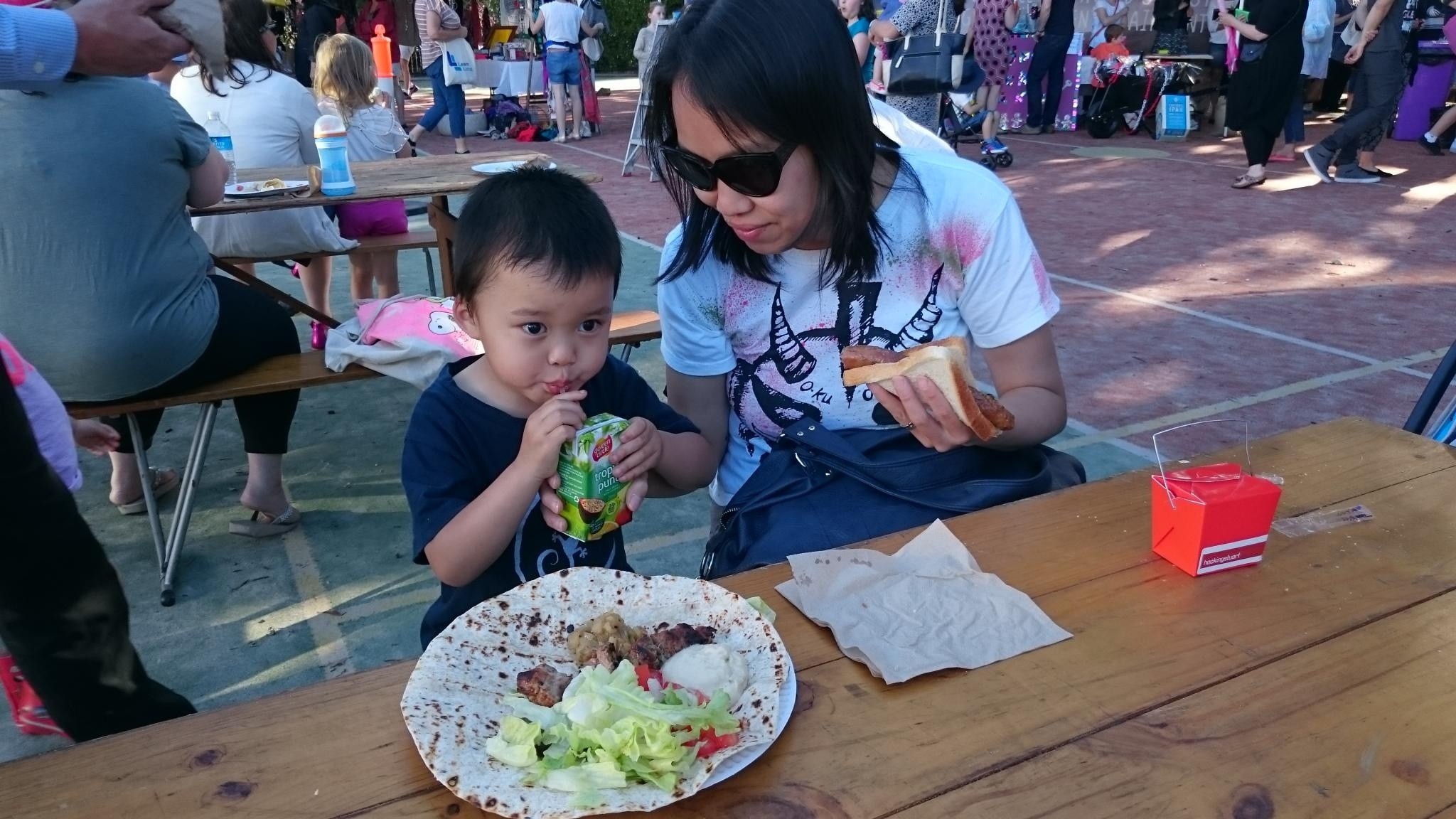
[1040,124,1053,133]
[1010,124,1041,134]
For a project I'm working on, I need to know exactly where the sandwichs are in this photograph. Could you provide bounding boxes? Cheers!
[842,339,1015,442]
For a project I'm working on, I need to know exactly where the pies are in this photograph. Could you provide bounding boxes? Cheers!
[400,566,789,819]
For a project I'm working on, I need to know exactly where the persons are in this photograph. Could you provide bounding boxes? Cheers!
[639,2,1075,585]
[265,0,1456,195]
[401,164,727,659]
[2,0,217,741]
[312,33,414,335]
[1,0,300,534]
[1,334,122,493]
[165,1,345,350]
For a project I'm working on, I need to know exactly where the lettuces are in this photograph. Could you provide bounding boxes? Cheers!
[485,645,749,795]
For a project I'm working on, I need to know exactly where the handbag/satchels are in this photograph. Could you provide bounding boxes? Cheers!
[578,120,592,138]
[883,0,967,94]
[534,120,559,141]
[697,416,1088,581]
[434,26,478,88]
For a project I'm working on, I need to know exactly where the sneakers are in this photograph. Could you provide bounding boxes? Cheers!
[989,136,1008,150]
[1303,143,1335,183]
[403,92,412,100]
[1417,135,1445,155]
[868,79,890,95]
[981,142,1005,154]
[1334,162,1381,183]
[409,84,418,95]
[865,84,875,98]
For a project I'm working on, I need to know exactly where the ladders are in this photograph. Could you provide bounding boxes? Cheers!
[621,18,678,184]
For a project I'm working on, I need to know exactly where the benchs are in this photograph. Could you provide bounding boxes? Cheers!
[211,227,441,307]
[69,308,666,607]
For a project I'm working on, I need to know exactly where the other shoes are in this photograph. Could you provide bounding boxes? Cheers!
[455,149,472,154]
[1330,116,1348,123]
[406,132,417,157]
[997,130,1008,134]
[550,136,565,142]
[566,135,580,140]
[1357,165,1393,177]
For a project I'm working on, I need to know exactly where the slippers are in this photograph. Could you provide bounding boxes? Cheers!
[1268,150,1297,161]
[1235,174,1247,182]
[1231,175,1266,188]
[228,505,298,538]
[118,467,180,515]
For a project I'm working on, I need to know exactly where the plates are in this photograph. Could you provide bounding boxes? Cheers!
[471,161,557,175]
[224,180,311,197]
[401,563,799,819]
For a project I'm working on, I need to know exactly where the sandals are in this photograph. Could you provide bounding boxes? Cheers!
[310,320,331,351]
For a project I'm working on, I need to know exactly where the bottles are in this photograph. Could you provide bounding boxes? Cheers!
[315,101,358,197]
[205,110,237,187]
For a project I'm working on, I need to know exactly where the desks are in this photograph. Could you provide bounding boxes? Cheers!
[470,58,551,112]
[1143,52,1213,137]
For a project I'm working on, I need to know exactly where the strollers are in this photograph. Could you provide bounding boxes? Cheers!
[937,93,1013,172]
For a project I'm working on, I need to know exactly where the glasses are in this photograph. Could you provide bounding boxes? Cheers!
[658,133,802,198]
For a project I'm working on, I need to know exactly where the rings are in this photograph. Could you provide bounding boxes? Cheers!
[899,421,915,432]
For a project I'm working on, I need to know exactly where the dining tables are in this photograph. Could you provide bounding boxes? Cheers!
[0,416,1456,819]
[188,148,562,298]
